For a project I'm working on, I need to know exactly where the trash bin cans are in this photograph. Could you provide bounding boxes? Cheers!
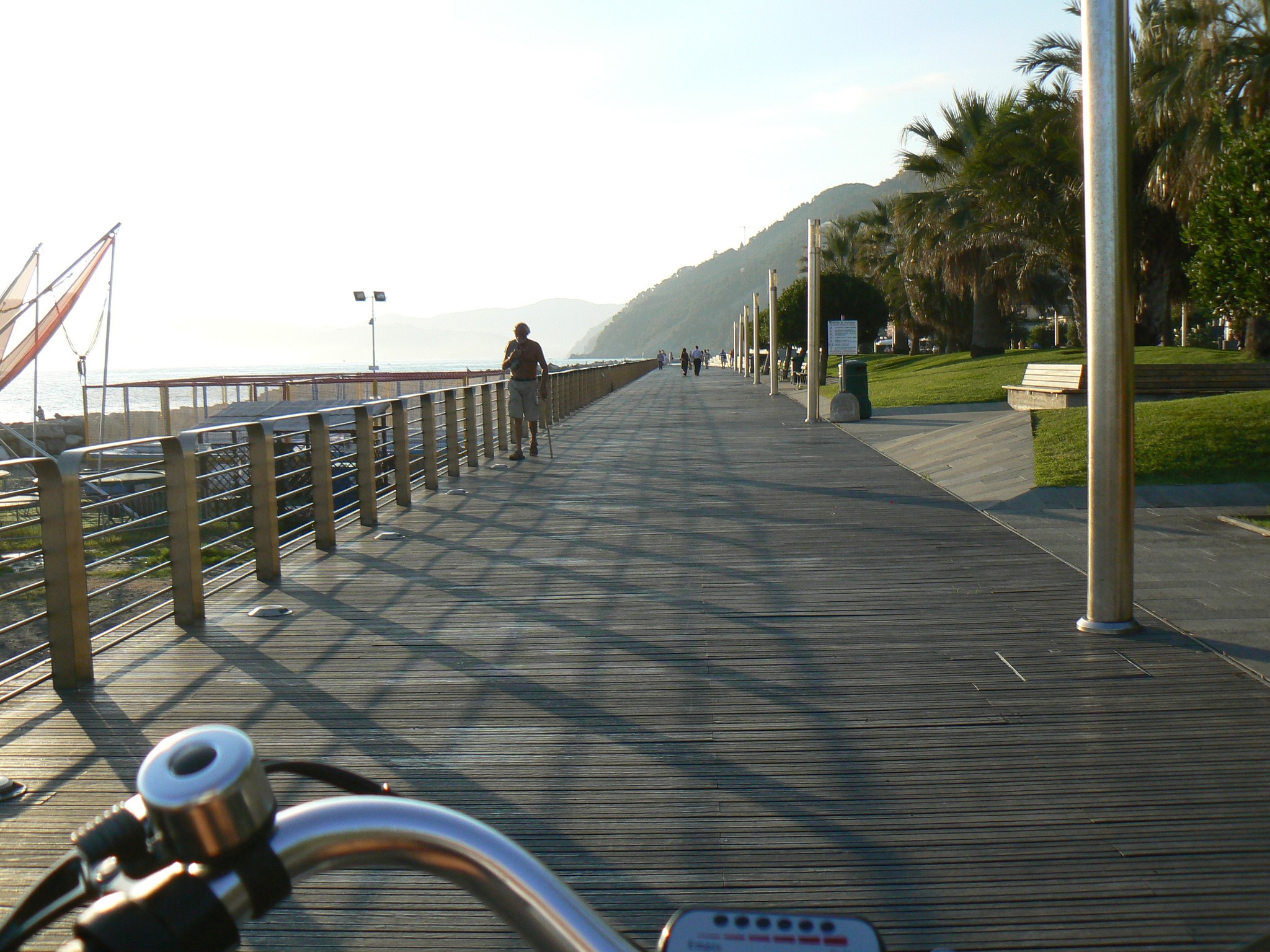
[836,360,871,420]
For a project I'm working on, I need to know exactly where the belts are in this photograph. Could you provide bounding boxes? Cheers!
[510,376,537,382]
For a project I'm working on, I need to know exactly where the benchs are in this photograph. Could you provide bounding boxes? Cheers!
[1001,363,1270,413]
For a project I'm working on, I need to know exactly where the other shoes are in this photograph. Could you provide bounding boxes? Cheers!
[530,437,538,456]
[509,450,525,460]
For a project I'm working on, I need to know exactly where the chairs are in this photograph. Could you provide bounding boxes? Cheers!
[769,359,807,389]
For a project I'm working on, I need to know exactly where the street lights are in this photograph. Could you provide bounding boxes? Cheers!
[354,290,386,399]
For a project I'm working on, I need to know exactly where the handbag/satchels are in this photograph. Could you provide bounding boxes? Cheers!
[689,361,692,370]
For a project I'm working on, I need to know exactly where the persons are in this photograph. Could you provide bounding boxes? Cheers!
[669,349,712,369]
[728,349,734,369]
[679,348,691,376]
[657,350,667,369]
[691,345,703,376]
[502,322,549,460]
[719,349,727,369]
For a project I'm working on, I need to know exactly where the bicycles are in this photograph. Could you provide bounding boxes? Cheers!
[0,722,892,952]
